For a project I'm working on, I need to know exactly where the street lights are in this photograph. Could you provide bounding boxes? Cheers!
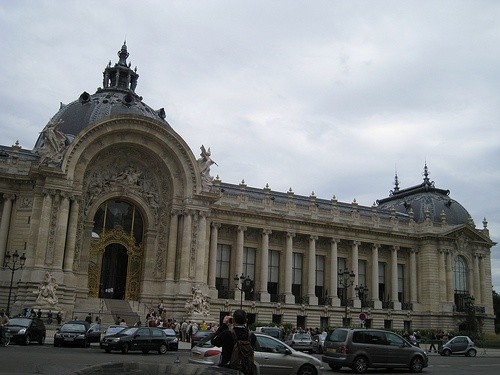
[233,273,253,310]
[339,267,357,326]
[355,284,369,327]
[4,250,27,317]
[464,295,475,335]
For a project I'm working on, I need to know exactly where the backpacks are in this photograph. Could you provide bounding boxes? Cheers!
[230,329,254,373]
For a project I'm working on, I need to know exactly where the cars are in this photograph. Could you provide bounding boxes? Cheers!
[189,332,324,375]
[440,336,478,358]
[158,327,178,351]
[191,330,214,349]
[101,325,128,341]
[285,333,313,354]
[4,317,46,345]
[100,326,179,354]
[53,321,101,347]
[262,328,279,339]
[313,334,327,353]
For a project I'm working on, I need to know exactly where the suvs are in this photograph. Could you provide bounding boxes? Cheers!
[322,328,428,374]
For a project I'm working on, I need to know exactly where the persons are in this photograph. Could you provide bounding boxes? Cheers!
[0,310,10,347]
[115,316,121,325]
[403,330,421,348]
[211,309,257,375]
[428,332,454,352]
[47,310,52,318]
[166,319,219,343]
[31,309,36,317]
[185,284,211,315]
[85,313,91,324]
[276,324,321,339]
[23,309,28,316]
[134,321,141,327]
[36,272,56,302]
[158,299,164,315]
[120,319,126,325]
[56,312,61,324]
[95,316,101,324]
[37,309,42,318]
[145,310,167,328]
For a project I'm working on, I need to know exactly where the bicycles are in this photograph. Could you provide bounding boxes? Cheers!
[1,327,11,347]
